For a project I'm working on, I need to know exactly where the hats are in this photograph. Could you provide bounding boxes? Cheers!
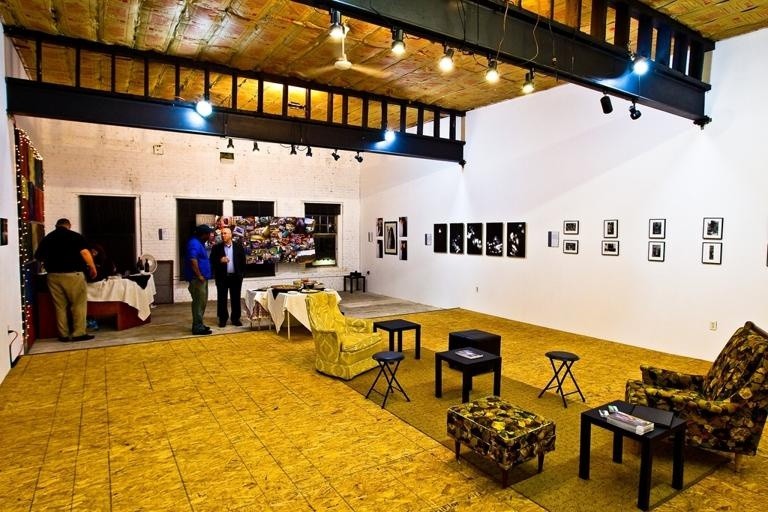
[196,225,212,233]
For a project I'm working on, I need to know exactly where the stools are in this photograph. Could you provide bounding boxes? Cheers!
[447,395,556,488]
[538,351,578,405]
[447,329,501,375]
[366,352,411,409]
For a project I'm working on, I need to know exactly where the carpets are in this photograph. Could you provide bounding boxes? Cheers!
[341,346,732,512]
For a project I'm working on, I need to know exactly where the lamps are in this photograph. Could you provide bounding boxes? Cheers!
[486,61,503,87]
[330,9,353,72]
[438,48,457,73]
[630,103,641,120]
[523,73,539,92]
[601,95,612,115]
[392,31,406,57]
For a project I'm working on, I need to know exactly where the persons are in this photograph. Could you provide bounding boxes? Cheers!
[204,231,216,248]
[33,218,98,342]
[182,225,213,335]
[209,228,246,328]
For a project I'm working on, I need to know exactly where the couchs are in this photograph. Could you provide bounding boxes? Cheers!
[304,291,386,381]
[626,321,768,473]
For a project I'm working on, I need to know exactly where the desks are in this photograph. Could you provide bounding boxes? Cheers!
[344,276,365,294]
[247,290,269,330]
[269,288,336,342]
[89,273,154,330]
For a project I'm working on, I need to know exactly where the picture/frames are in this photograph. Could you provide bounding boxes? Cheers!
[564,220,579,233]
[383,221,399,256]
[648,241,665,262]
[603,241,618,256]
[649,219,665,238]
[564,240,578,253]
[604,219,618,238]
[702,242,722,264]
[704,218,724,239]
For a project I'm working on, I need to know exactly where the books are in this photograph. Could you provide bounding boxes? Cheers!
[455,349,484,360]
[608,411,655,435]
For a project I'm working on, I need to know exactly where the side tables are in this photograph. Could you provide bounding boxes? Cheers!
[579,400,688,511]
[373,318,422,360]
[435,347,499,403]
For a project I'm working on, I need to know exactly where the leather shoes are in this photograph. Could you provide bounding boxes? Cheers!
[218,322,226,327]
[72,334,95,341]
[232,321,242,326]
[58,337,70,342]
[192,330,212,335]
